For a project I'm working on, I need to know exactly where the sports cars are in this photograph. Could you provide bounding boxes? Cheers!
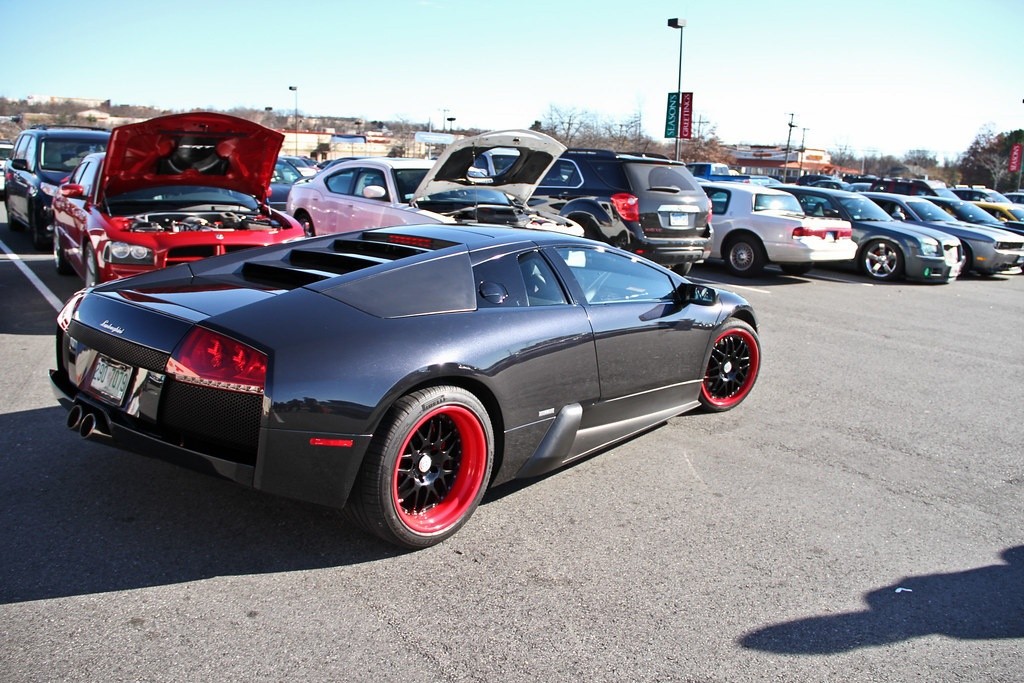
[47,221,764,549]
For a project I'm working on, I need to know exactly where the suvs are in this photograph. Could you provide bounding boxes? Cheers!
[5,124,115,252]
[525,146,716,277]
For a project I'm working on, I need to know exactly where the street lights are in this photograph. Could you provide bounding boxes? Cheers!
[665,16,687,161]
[289,86,298,157]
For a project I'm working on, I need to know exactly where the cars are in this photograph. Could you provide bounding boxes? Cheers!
[47,108,309,289]
[699,180,859,280]
[0,136,1024,201]
[765,185,965,283]
[854,191,1024,278]
[284,126,586,236]
